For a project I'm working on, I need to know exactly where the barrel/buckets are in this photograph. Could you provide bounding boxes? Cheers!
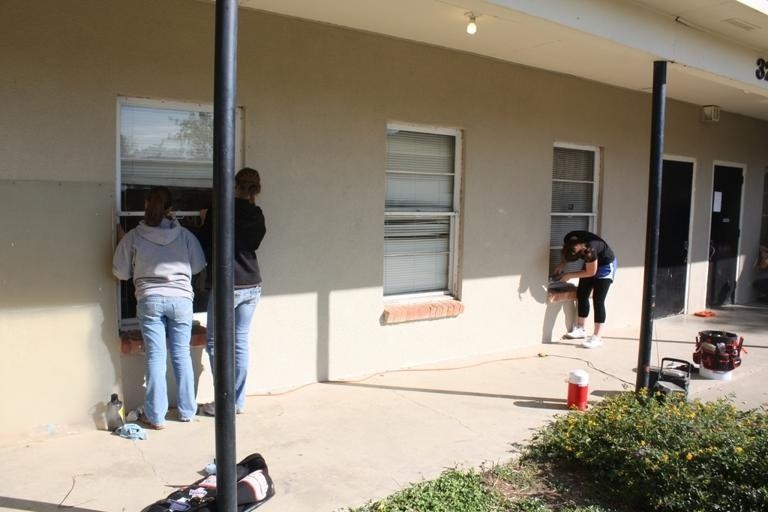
[698,330,739,381]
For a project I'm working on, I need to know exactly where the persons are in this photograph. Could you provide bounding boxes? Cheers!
[200,167,267,416]
[112,187,207,429]
[554,230,616,349]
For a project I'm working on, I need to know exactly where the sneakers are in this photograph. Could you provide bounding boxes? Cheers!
[582,334,601,348]
[202,402,215,417]
[138,412,168,430]
[564,325,585,338]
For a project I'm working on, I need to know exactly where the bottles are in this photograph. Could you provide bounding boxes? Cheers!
[105,393,144,431]
[567,369,588,410]
